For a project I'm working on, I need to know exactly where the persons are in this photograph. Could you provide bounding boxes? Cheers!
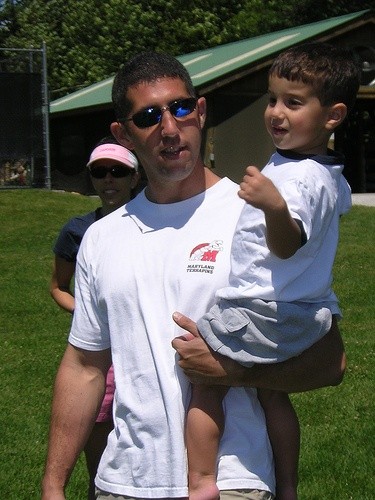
[182,45,359,500]
[6,162,26,185]
[48,134,140,500]
[41,51,342,500]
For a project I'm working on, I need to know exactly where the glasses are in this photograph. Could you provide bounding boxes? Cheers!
[90,163,132,179]
[121,96,201,130]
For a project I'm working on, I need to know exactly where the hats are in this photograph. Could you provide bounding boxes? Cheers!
[86,143,139,171]
